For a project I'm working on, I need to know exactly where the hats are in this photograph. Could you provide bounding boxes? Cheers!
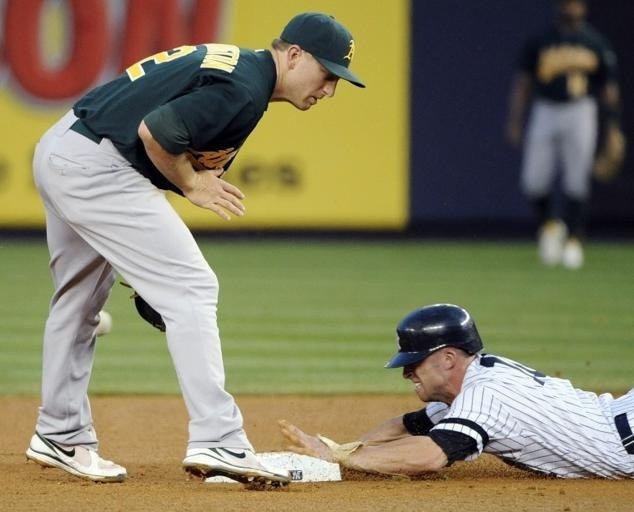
[278,9,370,93]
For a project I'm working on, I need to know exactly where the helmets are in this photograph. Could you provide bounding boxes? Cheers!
[379,300,486,371]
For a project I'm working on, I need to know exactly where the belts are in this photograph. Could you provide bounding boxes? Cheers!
[66,116,104,151]
[611,412,634,458]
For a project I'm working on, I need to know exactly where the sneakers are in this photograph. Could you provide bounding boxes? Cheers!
[176,438,294,493]
[21,425,129,487]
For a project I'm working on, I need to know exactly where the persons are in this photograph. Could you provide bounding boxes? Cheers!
[21,12,366,488]
[274,300,634,479]
[504,1,626,268]
